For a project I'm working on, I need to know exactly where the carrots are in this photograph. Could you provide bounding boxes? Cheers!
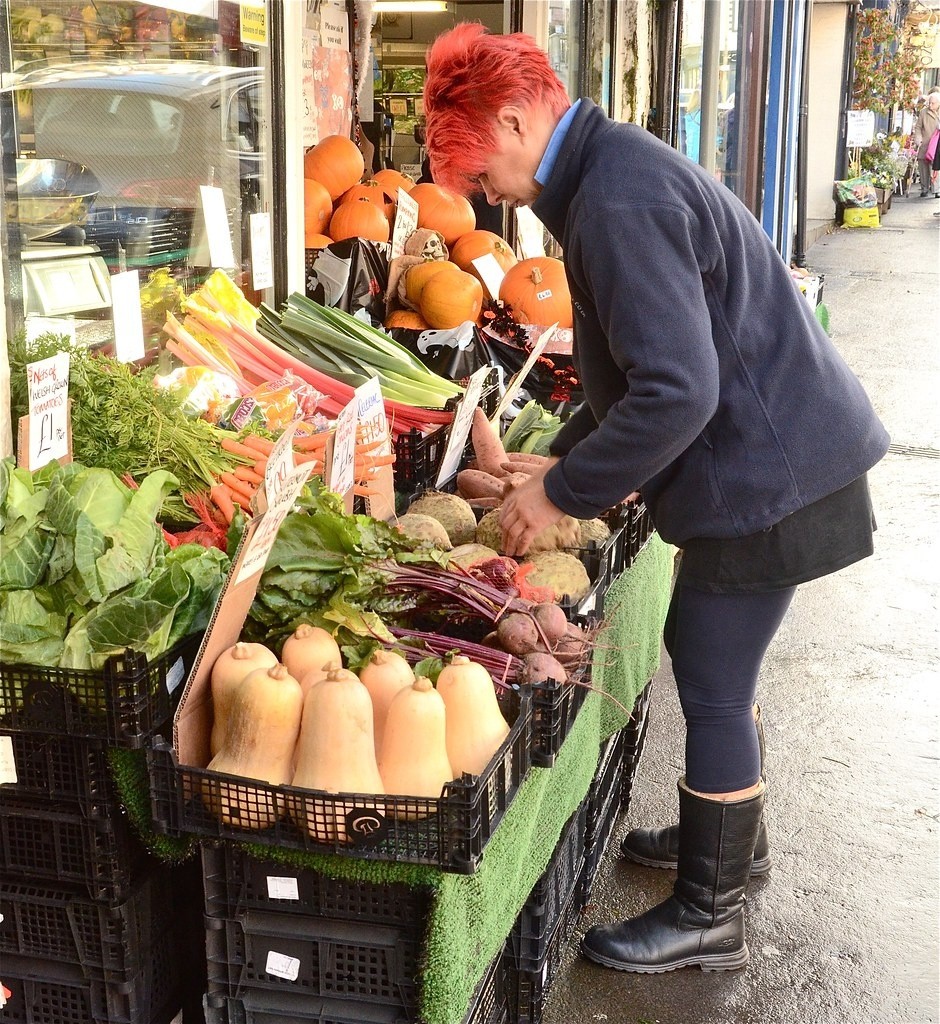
[212,427,396,526]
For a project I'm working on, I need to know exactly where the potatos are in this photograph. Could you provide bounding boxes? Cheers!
[457,408,549,508]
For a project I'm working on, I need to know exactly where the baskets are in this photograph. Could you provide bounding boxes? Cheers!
[0,367,654,1024]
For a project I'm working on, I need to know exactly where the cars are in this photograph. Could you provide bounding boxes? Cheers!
[676,86,771,150]
[12,57,267,275]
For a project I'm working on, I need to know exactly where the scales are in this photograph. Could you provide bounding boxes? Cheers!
[1,158,113,318]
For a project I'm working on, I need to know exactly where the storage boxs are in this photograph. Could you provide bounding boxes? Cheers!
[842,165,913,230]
[799,271,825,311]
[0,380,668,1024]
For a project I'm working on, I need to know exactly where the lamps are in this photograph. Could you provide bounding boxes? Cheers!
[371,1,456,14]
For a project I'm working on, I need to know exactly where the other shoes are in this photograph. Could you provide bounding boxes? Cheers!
[935,192,940,198]
[921,192,928,197]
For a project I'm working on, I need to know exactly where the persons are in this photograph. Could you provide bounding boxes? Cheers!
[914,87,940,217]
[426,23,891,974]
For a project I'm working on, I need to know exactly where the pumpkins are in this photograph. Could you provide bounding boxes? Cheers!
[303,136,574,331]
[201,625,511,843]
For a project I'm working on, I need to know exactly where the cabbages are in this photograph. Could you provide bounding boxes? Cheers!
[0,453,231,720]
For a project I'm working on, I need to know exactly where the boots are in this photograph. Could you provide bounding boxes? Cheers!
[581,777,762,974]
[621,704,773,876]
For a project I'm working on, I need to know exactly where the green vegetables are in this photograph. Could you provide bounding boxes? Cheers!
[374,65,429,133]
